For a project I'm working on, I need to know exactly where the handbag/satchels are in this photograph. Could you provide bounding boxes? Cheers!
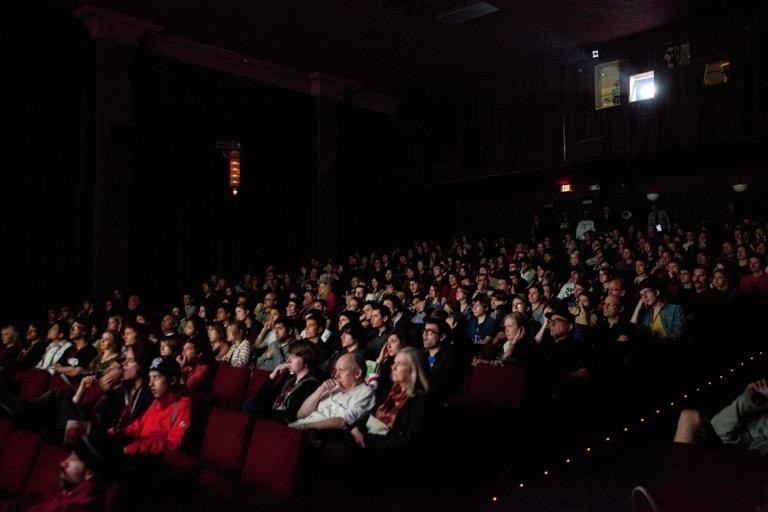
[240,395,256,413]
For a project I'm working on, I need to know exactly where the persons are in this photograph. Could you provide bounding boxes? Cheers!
[663,46,675,69]
[600,81,621,108]
[721,61,731,82]
[675,44,690,65]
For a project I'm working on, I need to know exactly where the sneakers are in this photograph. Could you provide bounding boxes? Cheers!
[631,486,659,512]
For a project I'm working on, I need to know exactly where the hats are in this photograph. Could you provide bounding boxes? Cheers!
[149,356,182,379]
[74,431,123,482]
[545,311,574,326]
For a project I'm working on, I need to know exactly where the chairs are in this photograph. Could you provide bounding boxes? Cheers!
[1,361,528,511]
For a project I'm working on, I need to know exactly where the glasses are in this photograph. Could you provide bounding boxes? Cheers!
[69,326,84,331]
[547,318,568,324]
[423,329,440,336]
